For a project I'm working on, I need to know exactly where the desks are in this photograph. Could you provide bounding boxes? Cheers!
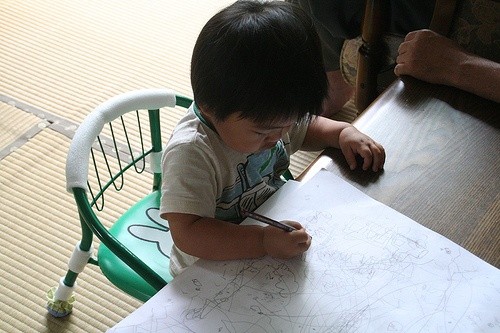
[103,39,500,332]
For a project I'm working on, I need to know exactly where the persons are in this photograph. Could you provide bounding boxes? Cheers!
[159,0,386,279]
[284,0,500,119]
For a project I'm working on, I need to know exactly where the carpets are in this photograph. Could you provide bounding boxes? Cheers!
[1,1,358,333]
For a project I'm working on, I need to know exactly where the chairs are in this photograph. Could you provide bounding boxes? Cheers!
[46,89,193,301]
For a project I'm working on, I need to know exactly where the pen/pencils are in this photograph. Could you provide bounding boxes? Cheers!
[242,210,312,241]
